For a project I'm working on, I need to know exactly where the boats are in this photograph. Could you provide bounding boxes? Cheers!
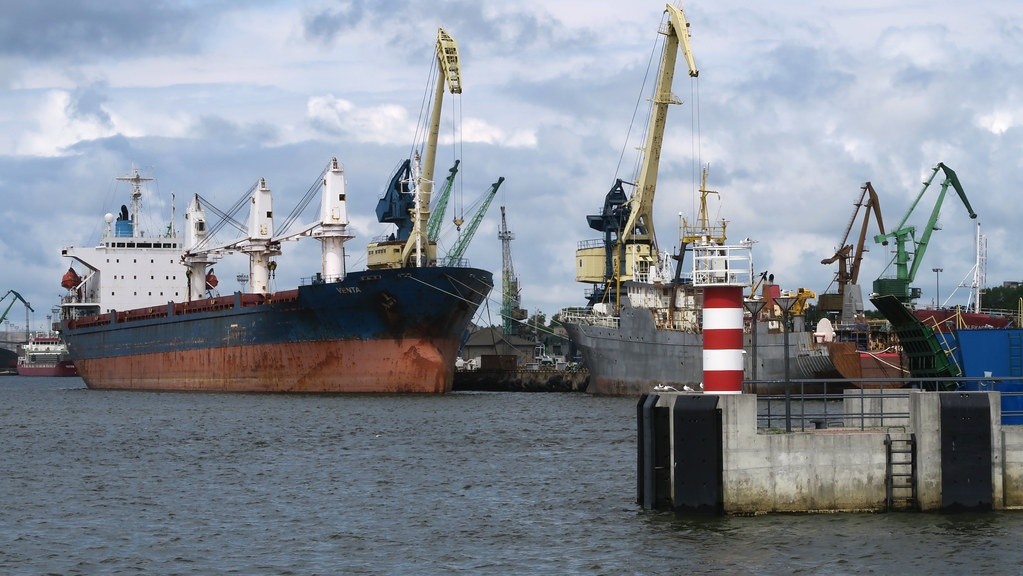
[419,159,589,391]
[51,25,493,394]
[552,2,886,398]
[866,161,1023,426]
[809,181,1023,389]
[16,316,81,377]
